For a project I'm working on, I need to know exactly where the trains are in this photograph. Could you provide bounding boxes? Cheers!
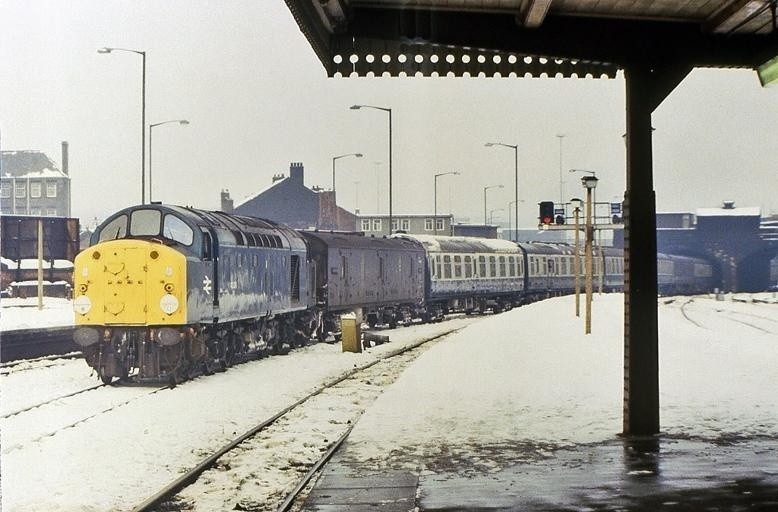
[74,202,713,384]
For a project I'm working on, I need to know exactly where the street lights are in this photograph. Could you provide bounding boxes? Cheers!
[565,167,600,333]
[555,133,565,203]
[484,142,525,242]
[333,104,392,235]
[434,172,461,235]
[97,47,190,204]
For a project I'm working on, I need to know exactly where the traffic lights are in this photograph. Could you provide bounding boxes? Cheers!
[539,201,554,224]
